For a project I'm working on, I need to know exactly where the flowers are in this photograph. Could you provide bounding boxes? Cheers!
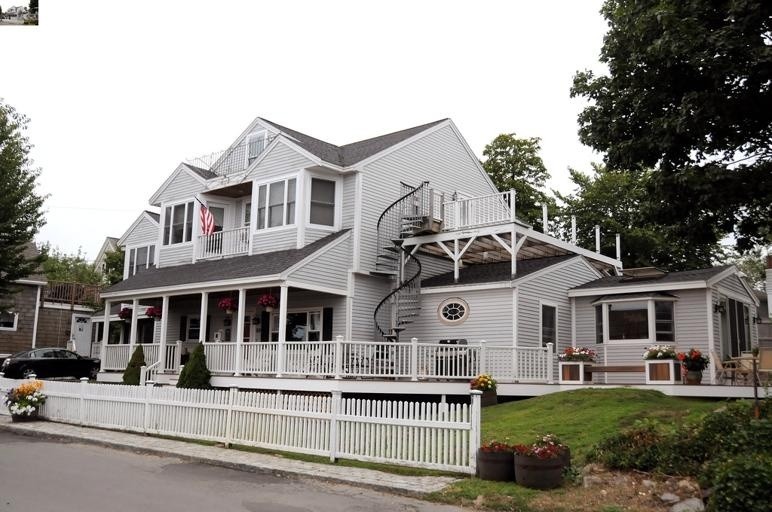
[145,306,162,319]
[256,294,277,306]
[640,344,710,371]
[470,374,496,391]
[1,379,48,416]
[557,345,599,365]
[477,430,568,459]
[118,308,132,319]
[217,297,238,311]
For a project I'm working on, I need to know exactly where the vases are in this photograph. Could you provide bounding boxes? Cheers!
[685,370,702,385]
[480,391,497,406]
[266,306,273,312]
[153,316,160,321]
[649,363,680,380]
[477,451,514,482]
[513,455,562,490]
[562,365,592,381]
[558,448,570,467]
[11,405,39,423]
[125,318,130,322]
[225,309,233,314]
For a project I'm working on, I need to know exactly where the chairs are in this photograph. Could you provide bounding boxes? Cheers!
[712,350,772,386]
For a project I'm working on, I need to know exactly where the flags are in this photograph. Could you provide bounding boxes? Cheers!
[200,204,215,237]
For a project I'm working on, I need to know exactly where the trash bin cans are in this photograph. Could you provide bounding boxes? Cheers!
[437,339,467,377]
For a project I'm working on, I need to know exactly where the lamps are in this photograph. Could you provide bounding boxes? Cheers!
[714,301,725,313]
[753,313,762,324]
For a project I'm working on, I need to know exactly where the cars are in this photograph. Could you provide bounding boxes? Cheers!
[1,348,101,380]
